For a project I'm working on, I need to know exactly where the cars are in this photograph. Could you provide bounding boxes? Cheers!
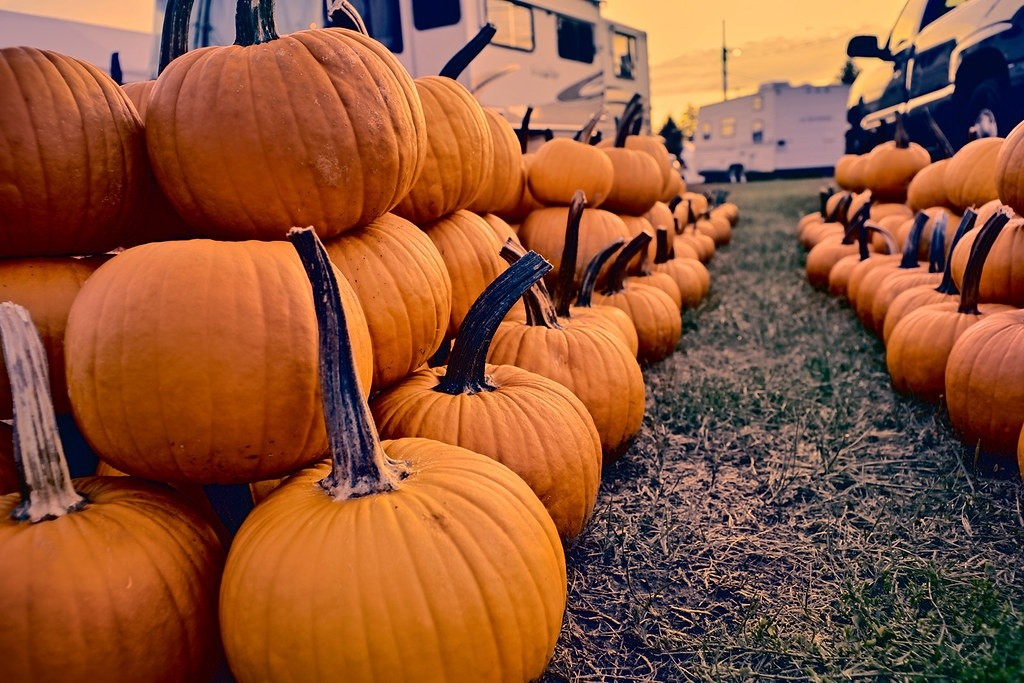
[844,0,1024,166]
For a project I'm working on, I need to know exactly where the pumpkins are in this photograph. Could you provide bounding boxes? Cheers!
[0,0,741,683]
[798,107,1024,484]
